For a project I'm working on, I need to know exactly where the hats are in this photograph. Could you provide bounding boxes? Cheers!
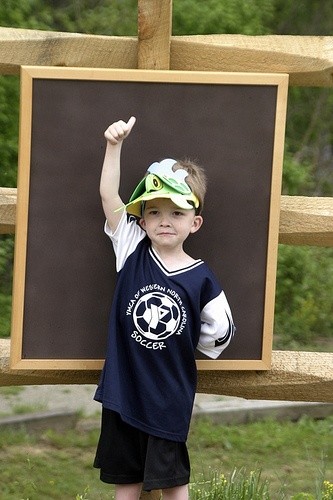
[124,158,200,218]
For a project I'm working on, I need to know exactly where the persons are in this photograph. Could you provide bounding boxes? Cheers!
[92,116,238,500]
